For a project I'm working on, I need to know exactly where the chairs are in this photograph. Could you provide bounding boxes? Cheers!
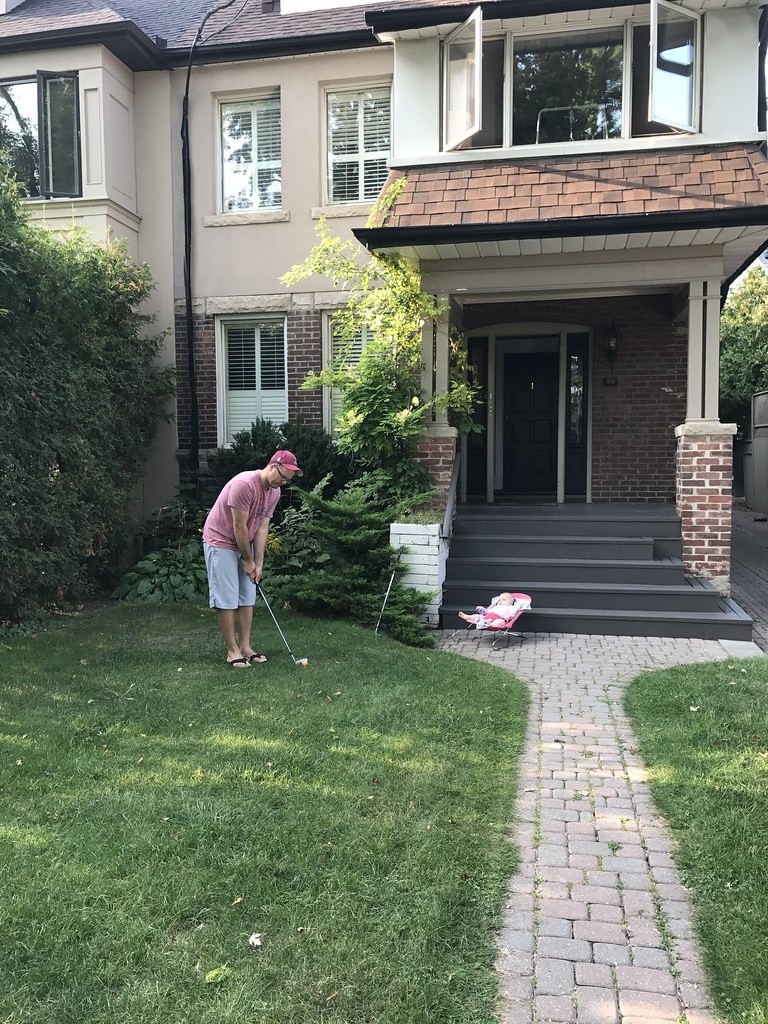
[451,592,533,651]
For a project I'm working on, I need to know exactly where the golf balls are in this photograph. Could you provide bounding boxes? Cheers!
[300,660,308,667]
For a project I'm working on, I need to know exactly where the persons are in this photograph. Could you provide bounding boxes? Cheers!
[459,592,516,626]
[203,451,302,667]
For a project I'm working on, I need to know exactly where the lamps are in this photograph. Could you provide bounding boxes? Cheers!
[602,321,619,386]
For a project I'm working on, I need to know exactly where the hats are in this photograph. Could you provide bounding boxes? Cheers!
[270,450,304,477]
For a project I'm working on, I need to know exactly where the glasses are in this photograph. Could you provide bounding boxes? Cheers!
[275,466,292,483]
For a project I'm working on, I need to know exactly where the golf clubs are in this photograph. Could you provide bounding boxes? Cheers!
[373,546,403,635]
[255,579,308,665]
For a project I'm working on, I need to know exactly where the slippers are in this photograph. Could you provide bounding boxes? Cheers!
[250,653,268,664]
[225,657,251,668]
